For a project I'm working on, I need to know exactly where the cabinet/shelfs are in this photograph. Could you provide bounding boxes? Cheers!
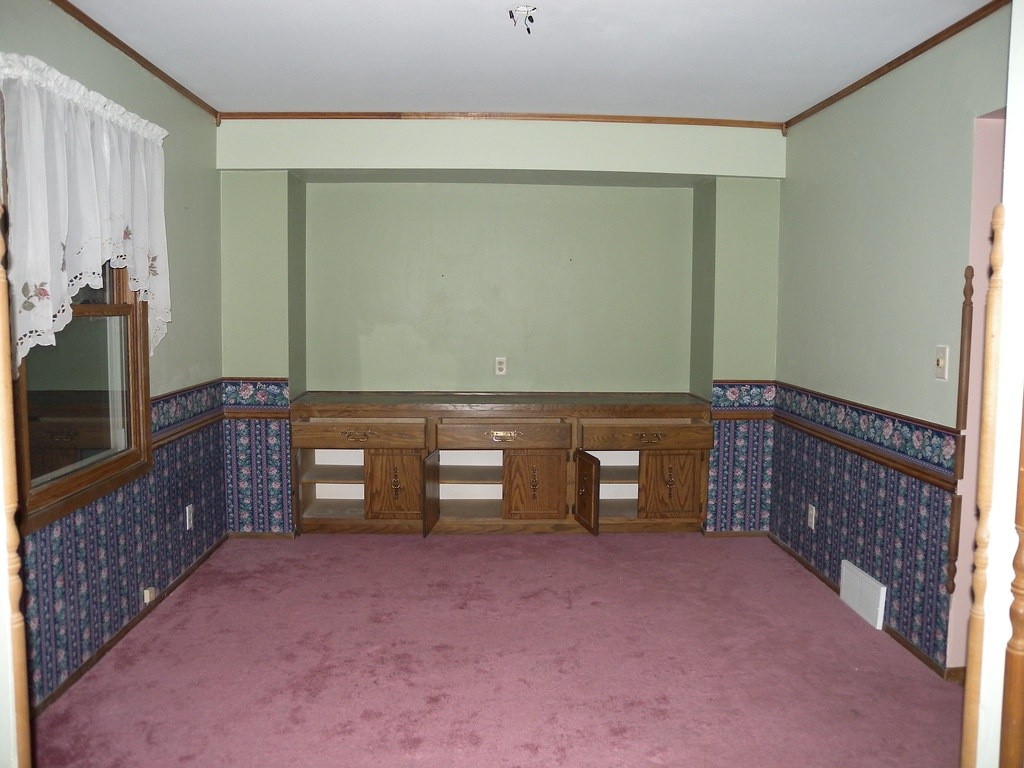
[291,392,714,537]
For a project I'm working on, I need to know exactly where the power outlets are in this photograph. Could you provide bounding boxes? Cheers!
[808,504,815,529]
[496,358,506,375]
[186,504,193,530]
[144,587,155,603]
